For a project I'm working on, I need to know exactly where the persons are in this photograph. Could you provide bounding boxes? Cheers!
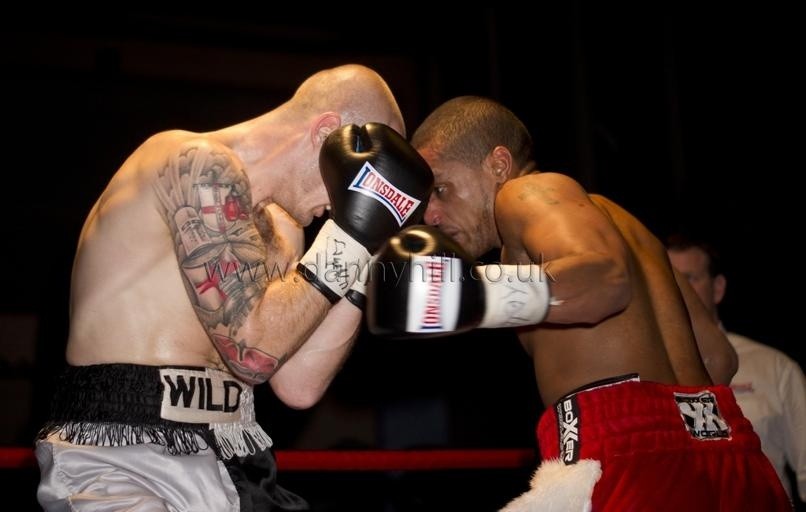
[367,95,791,511]
[660,234,805,512]
[35,63,436,511]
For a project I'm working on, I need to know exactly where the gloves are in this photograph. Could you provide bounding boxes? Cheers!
[297,122,435,305]
[367,224,551,340]
